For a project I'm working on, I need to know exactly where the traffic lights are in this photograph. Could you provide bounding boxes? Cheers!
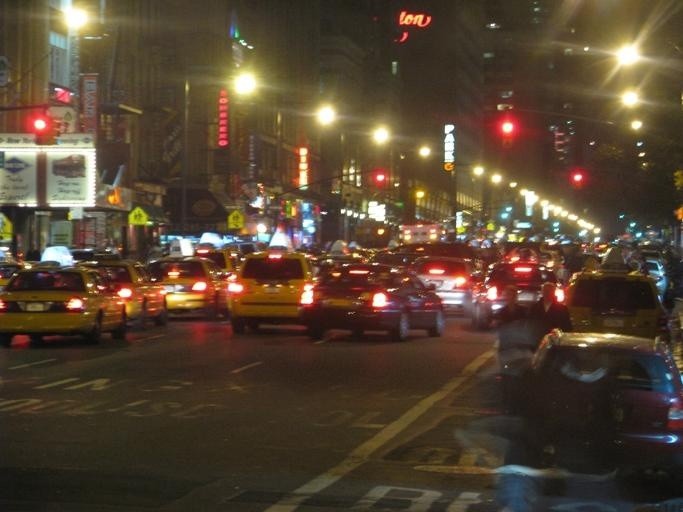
[553,129,565,154]
[375,170,384,185]
[571,168,581,188]
[32,115,46,134]
[500,118,512,150]
